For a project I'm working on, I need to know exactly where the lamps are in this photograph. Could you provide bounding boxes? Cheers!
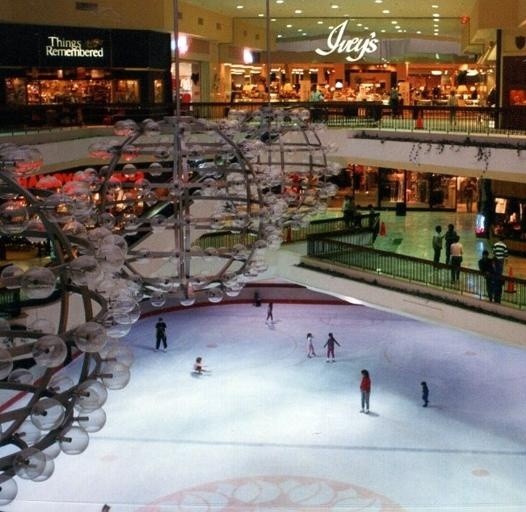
[2,133,148,505]
[213,103,341,238]
[84,111,292,313]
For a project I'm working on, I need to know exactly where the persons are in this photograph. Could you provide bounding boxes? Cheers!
[266,301,273,320]
[389,84,404,119]
[478,236,508,303]
[308,84,325,124]
[420,381,429,407]
[463,179,477,213]
[323,332,341,362]
[448,90,458,130]
[192,356,208,373]
[155,317,168,353]
[307,333,316,359]
[254,290,261,307]
[433,224,464,283]
[342,195,375,228]
[359,369,371,414]
